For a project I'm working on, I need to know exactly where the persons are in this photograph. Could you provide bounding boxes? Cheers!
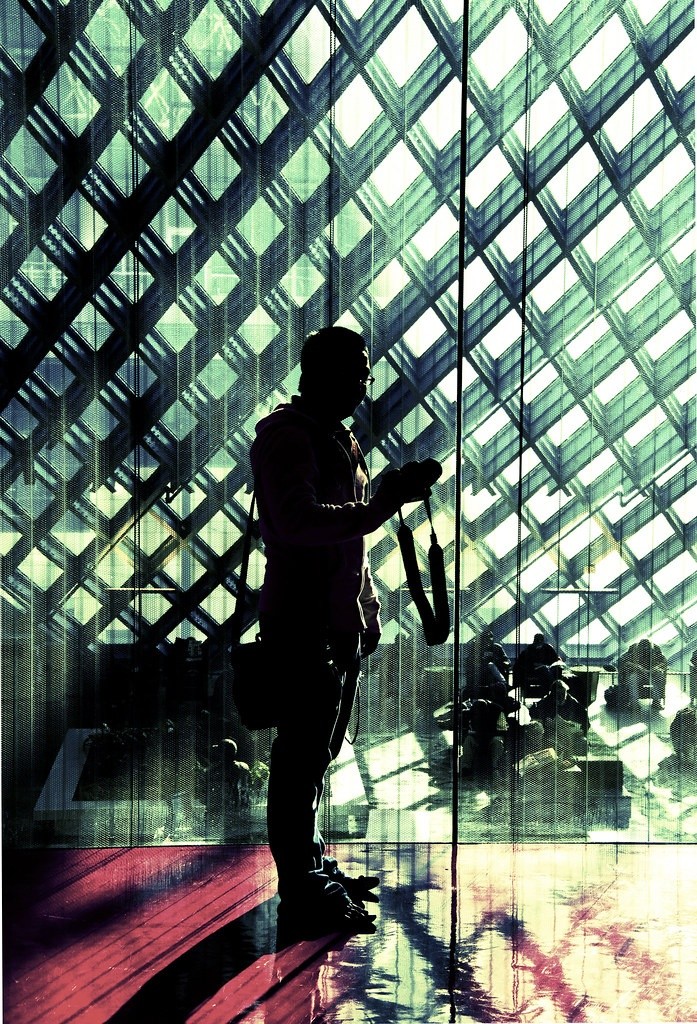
[249,327,425,934]
[180,638,204,674]
[480,630,510,685]
[532,680,590,735]
[462,699,509,776]
[622,640,662,711]
[518,722,576,769]
[205,738,249,806]
[525,634,558,695]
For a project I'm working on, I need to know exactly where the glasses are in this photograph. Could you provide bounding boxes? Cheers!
[315,366,375,387]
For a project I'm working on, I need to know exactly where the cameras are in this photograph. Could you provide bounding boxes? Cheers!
[394,458,443,504]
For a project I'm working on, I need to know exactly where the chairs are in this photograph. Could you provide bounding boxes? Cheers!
[619,650,668,707]
[518,653,565,706]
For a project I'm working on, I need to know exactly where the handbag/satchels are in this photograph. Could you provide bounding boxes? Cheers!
[231,638,281,730]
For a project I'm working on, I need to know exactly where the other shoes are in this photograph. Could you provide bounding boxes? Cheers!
[652,699,663,710]
[335,875,379,894]
[277,893,376,928]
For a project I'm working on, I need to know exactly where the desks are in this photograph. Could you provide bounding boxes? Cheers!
[568,729,624,797]
[570,666,601,704]
